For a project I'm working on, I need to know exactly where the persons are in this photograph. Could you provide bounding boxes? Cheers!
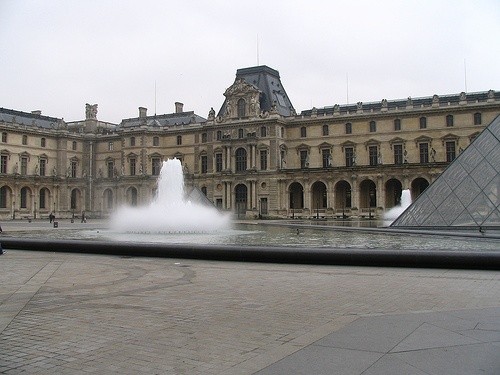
[52,215,55,222]
[80,212,86,223]
[49,213,53,223]
[72,213,75,220]
